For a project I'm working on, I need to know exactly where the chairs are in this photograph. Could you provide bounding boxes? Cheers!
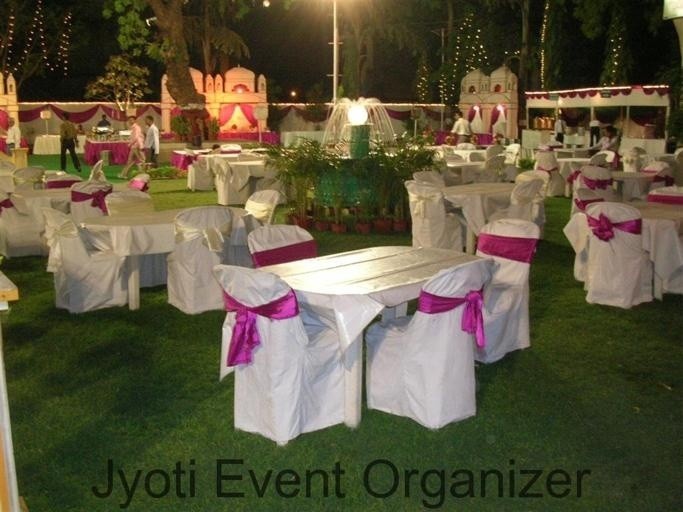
[247,224,318,269]
[474,217,541,365]
[364,255,494,431]
[209,265,363,449]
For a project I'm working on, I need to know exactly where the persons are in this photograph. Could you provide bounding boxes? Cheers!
[590,120,601,147]
[142,115,160,164]
[76,125,85,135]
[117,114,146,180]
[190,118,203,150]
[554,114,567,143]
[5,116,21,156]
[97,115,113,127]
[57,112,80,173]
[450,112,472,146]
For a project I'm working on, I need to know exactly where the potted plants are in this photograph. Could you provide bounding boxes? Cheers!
[251,132,450,236]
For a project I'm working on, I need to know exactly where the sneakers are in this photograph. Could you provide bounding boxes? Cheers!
[117,173,128,179]
